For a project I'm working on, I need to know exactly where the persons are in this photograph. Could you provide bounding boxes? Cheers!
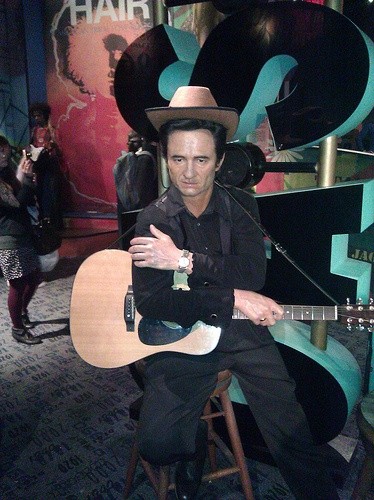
[112,131,158,252]
[0,135,44,345]
[27,101,51,148]
[127,86,343,500]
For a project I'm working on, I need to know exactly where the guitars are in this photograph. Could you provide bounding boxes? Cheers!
[70,250,374,368]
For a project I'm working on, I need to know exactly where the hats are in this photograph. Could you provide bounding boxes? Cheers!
[146,86,241,142]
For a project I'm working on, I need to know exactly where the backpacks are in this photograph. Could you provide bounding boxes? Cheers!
[114,151,157,213]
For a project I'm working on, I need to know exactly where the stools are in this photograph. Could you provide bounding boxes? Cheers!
[129,364,254,500]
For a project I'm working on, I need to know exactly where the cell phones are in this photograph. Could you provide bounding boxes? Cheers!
[22,149,27,161]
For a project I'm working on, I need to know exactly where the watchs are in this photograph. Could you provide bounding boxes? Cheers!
[176,250,190,273]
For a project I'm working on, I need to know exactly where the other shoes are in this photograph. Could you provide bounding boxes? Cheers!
[11,313,42,344]
[174,420,209,500]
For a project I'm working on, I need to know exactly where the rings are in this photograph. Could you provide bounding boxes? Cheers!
[26,167,29,170]
[260,318,266,321]
